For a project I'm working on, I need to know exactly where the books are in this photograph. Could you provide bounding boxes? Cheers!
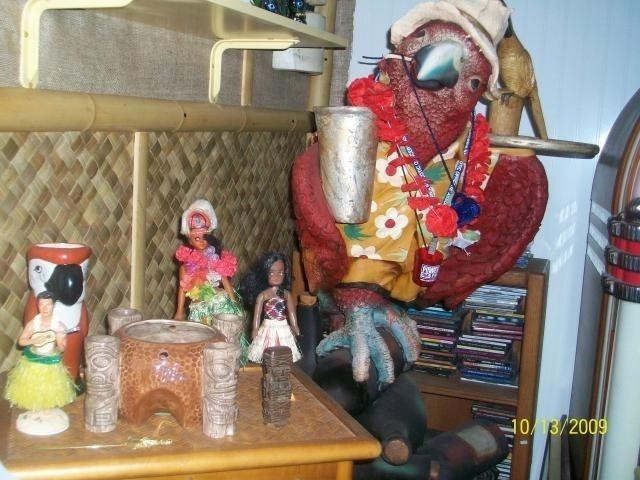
[404,286,528,388]
[472,404,517,479]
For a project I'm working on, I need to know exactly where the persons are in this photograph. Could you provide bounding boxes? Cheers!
[174,199,248,367]
[3,292,81,437]
[247,251,302,364]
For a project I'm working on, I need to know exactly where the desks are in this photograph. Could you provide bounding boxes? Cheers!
[0,359,382,479]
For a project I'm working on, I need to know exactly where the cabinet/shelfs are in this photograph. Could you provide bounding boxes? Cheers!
[291,249,549,479]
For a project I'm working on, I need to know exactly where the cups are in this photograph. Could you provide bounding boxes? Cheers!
[311,105,380,223]
